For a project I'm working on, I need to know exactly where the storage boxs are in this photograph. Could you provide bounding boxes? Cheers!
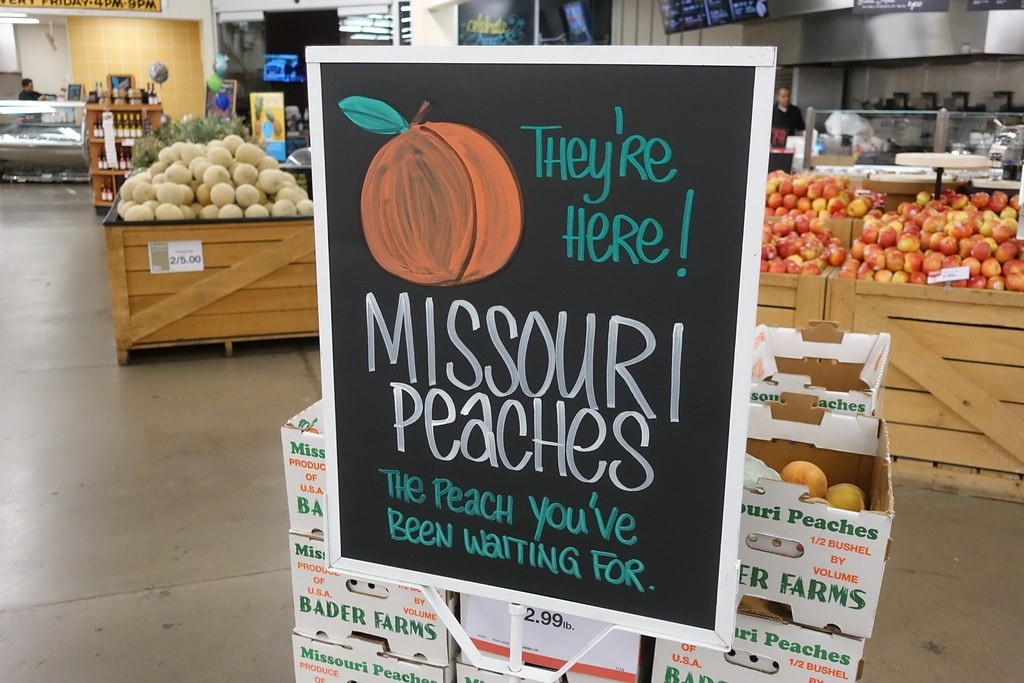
[279,323,894,682]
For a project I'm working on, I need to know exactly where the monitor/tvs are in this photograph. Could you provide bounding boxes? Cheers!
[68,84,81,100]
[106,75,133,93]
[559,0,596,45]
[263,54,300,84]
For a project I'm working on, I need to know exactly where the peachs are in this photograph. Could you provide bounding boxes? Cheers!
[780,460,866,512]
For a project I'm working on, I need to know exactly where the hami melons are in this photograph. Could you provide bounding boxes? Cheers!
[117,134,313,221]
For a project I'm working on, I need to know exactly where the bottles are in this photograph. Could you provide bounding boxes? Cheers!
[147,83,158,105]
[93,113,142,201]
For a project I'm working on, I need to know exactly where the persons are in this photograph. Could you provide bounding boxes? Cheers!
[769,89,805,172]
[283,60,293,80]
[19,79,46,123]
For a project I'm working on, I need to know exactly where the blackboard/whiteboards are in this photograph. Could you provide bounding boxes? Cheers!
[302,43,775,653]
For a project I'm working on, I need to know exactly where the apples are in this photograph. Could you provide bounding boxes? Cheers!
[759,169,1024,294]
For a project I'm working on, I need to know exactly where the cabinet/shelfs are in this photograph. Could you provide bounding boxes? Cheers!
[86,102,163,216]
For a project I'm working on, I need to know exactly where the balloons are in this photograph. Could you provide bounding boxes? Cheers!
[207,75,223,93]
[213,55,228,75]
[149,62,169,84]
[213,92,230,111]
